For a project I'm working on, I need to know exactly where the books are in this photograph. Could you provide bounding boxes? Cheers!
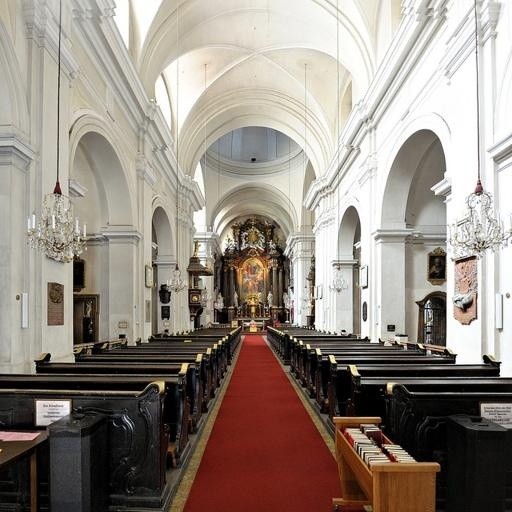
[343,422,418,475]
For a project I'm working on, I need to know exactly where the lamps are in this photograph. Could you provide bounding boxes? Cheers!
[25,0,89,263]
[300,62,313,310]
[447,0,512,259]
[165,1,186,295]
[213,137,226,310]
[328,0,349,293]
[196,64,214,309]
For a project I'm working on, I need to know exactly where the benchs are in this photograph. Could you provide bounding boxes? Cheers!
[265,323,512,512]
[0,327,245,512]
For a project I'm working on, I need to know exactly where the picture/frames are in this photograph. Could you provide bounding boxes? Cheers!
[145,264,154,288]
[73,254,86,292]
[360,266,368,289]
[428,247,447,285]
